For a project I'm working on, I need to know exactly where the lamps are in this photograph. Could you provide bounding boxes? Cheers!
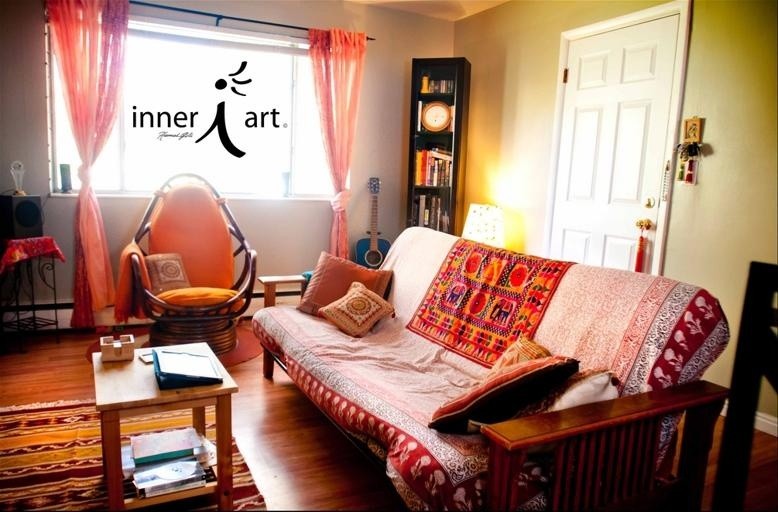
[462,202,504,249]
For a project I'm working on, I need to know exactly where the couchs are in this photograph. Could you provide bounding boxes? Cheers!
[254,228,732,512]
[131,174,256,355]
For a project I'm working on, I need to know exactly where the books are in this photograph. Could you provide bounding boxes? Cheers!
[415,147,454,187]
[418,193,441,231]
[449,106,455,133]
[428,79,454,94]
[417,101,422,132]
[131,428,207,499]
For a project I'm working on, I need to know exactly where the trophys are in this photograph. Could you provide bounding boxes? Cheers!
[8,160,26,197]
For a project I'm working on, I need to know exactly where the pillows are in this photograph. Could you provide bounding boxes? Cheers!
[142,253,192,296]
[299,251,394,318]
[485,334,550,373]
[511,364,619,422]
[317,281,392,336]
[430,353,581,433]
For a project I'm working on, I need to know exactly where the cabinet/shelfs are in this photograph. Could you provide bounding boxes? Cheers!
[406,57,472,235]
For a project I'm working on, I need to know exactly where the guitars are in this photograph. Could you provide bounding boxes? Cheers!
[355,178,391,268]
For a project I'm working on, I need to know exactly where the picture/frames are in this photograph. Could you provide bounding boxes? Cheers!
[683,119,702,142]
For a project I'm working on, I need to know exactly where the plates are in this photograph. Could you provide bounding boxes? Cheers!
[421,102,452,133]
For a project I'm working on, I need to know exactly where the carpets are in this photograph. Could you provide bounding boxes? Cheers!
[1,399,268,511]
